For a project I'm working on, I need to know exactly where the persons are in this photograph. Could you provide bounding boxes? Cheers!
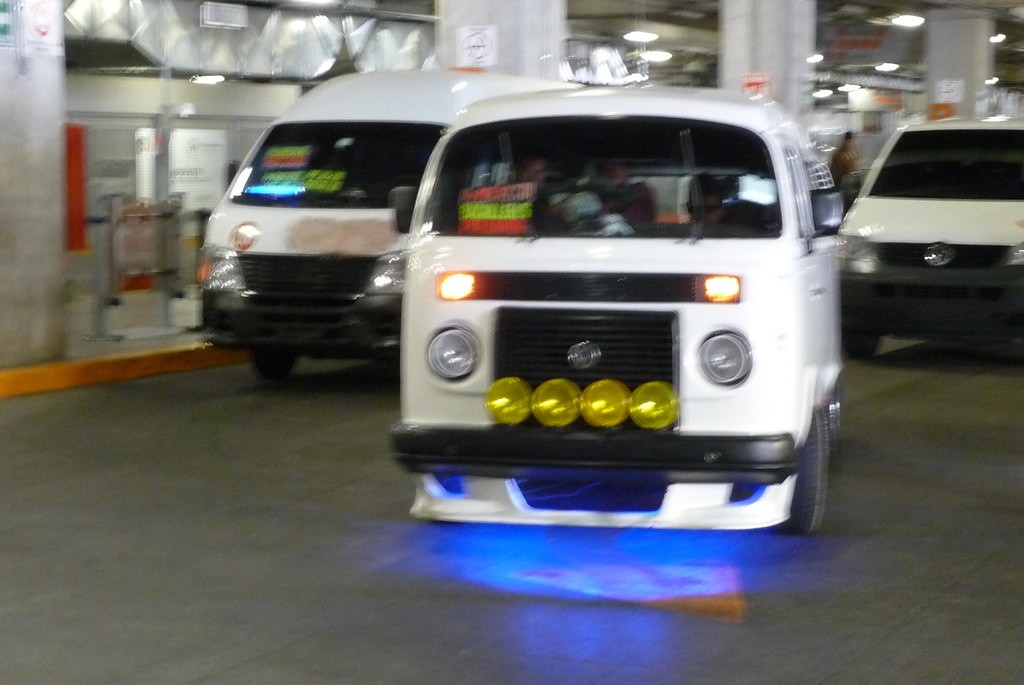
[685,174,727,227]
[583,156,655,224]
[831,131,857,172]
[522,150,573,220]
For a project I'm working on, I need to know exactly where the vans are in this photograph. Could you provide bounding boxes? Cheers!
[387,86,843,539]
[191,76,583,390]
[833,117,1023,363]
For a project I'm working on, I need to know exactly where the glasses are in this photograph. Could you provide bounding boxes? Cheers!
[689,205,722,213]
